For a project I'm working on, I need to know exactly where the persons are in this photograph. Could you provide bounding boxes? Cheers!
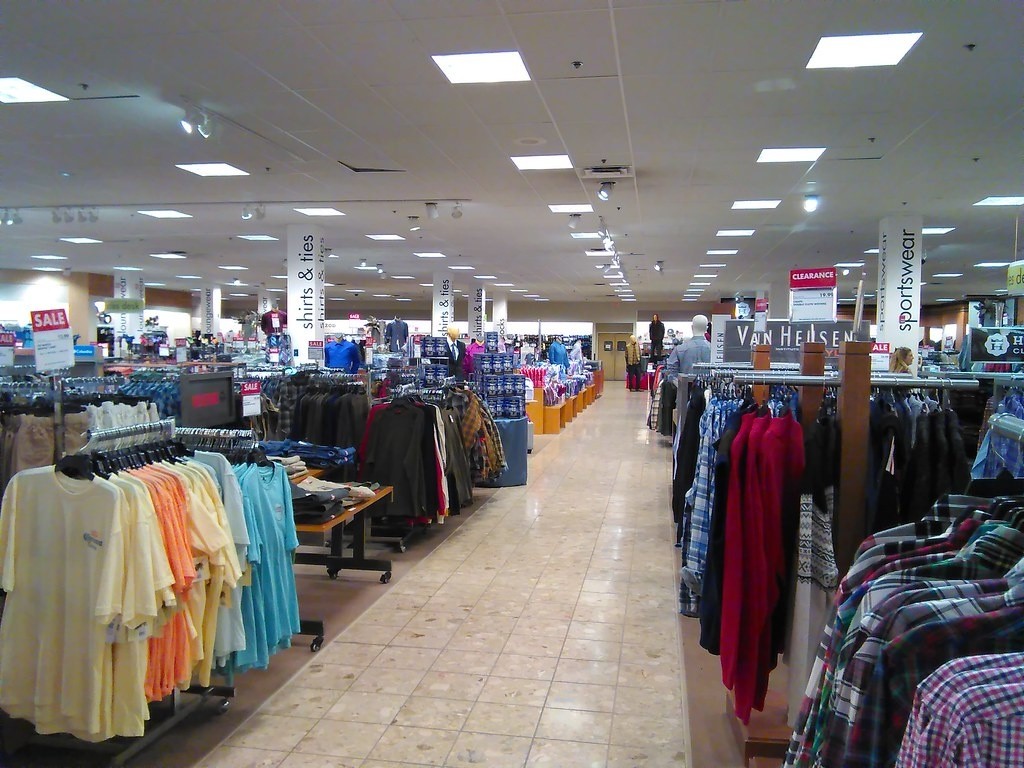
[462,334,487,380]
[669,313,711,379]
[261,304,287,335]
[625,335,644,392]
[444,326,465,380]
[888,346,914,374]
[650,314,665,362]
[568,340,584,374]
[384,316,408,353]
[548,336,570,372]
[325,335,360,375]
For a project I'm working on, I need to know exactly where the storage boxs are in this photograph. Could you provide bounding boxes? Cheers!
[419,331,526,419]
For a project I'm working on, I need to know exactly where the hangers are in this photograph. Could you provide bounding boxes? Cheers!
[0,363,190,417]
[235,362,466,410]
[694,361,1024,534]
[53,419,276,485]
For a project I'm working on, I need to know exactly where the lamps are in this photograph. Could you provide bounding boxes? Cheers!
[408,216,419,231]
[252,202,265,220]
[0,205,98,226]
[598,220,627,293]
[425,202,438,219]
[180,106,196,134]
[735,295,743,303]
[241,203,251,219]
[568,214,580,229]
[451,201,462,218]
[841,268,849,275]
[197,110,211,138]
[596,182,614,201]
[654,260,663,271]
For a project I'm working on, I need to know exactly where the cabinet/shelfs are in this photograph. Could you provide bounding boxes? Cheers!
[289,466,393,584]
[523,368,604,436]
